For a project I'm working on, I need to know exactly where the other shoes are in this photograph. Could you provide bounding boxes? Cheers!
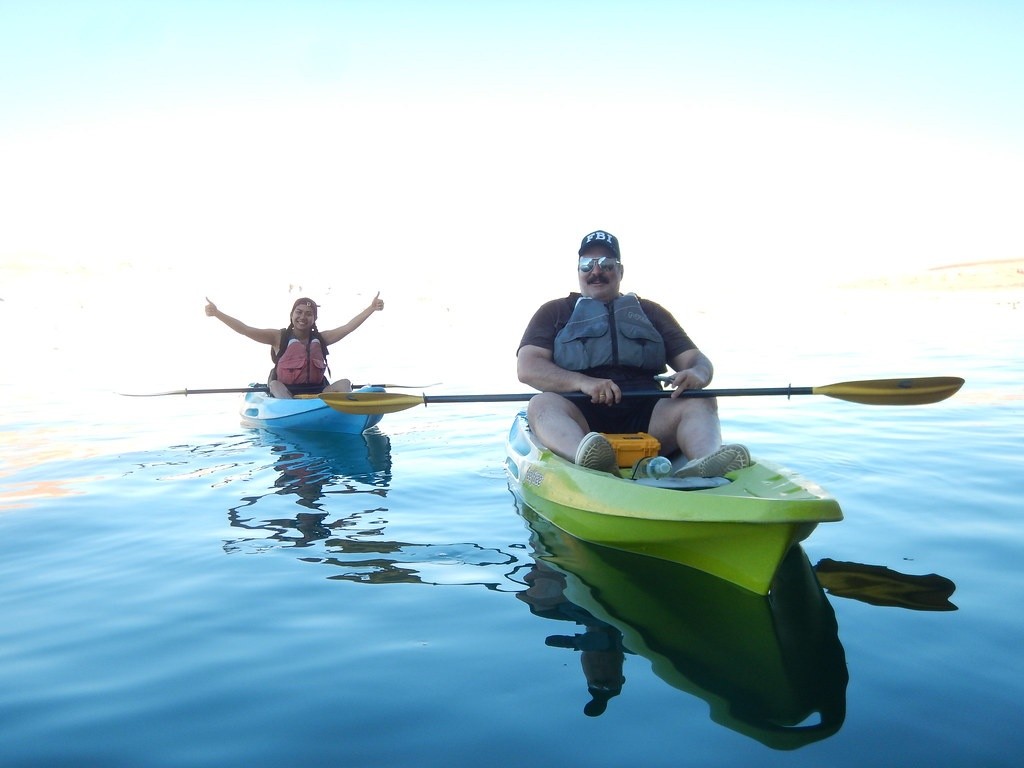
[323,379,350,392]
[268,380,295,399]
[672,443,751,479]
[574,432,623,478]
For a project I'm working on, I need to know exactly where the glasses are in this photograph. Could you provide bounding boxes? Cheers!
[579,256,618,272]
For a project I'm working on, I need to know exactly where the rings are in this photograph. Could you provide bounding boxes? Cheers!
[599,394,606,398]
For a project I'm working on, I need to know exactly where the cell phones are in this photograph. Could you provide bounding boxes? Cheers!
[653,375,675,384]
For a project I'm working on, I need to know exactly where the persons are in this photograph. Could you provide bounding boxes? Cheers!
[205,291,384,400]
[516,231,750,478]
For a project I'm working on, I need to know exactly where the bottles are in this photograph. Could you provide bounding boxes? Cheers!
[632,457,672,480]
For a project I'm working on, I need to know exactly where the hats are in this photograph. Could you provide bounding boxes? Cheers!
[293,298,320,313]
[578,230,620,259]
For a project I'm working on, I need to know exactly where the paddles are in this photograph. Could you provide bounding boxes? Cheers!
[118,382,443,398]
[318,375,966,416]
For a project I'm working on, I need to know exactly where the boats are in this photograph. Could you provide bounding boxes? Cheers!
[514,500,852,755]
[503,409,844,593]
[241,381,386,434]
